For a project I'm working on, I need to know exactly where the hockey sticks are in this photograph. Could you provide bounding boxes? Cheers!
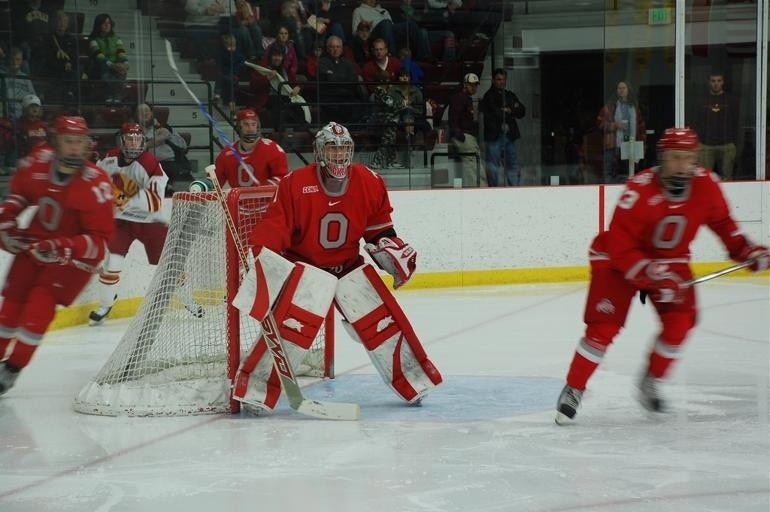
[206,163,360,420]
[685,257,758,286]
[165,39,262,186]
[10,236,110,274]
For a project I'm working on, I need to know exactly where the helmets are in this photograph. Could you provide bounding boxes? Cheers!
[314,121,354,179]
[233,109,260,142]
[120,123,146,158]
[56,116,91,169]
[658,127,699,190]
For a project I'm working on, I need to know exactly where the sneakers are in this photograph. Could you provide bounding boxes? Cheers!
[639,374,662,413]
[89,295,117,321]
[557,385,582,418]
[185,302,205,318]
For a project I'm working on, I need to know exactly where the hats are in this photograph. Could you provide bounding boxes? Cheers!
[23,94,41,107]
[465,73,480,84]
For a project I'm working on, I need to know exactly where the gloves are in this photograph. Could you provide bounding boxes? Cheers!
[735,246,770,271]
[0,228,33,253]
[634,264,684,308]
[31,237,71,266]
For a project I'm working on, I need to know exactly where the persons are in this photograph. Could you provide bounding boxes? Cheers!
[0,116,114,397]
[88,122,206,326]
[595,80,646,184]
[188,108,289,304]
[554,127,769,426]
[2,2,187,186]
[185,1,526,186]
[698,70,738,181]
[231,121,444,421]
